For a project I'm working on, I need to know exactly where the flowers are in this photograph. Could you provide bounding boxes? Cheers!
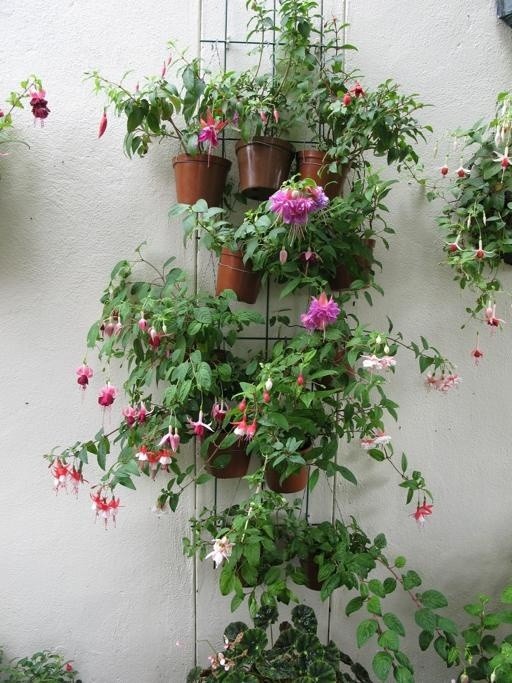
[428,83,510,371]
[42,284,465,537]
[77,6,422,171]
[0,71,51,162]
[229,179,401,305]
[189,537,250,679]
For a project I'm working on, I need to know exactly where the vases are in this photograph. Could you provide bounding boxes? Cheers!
[231,132,296,198]
[213,243,266,310]
[168,148,235,210]
[296,147,353,199]
